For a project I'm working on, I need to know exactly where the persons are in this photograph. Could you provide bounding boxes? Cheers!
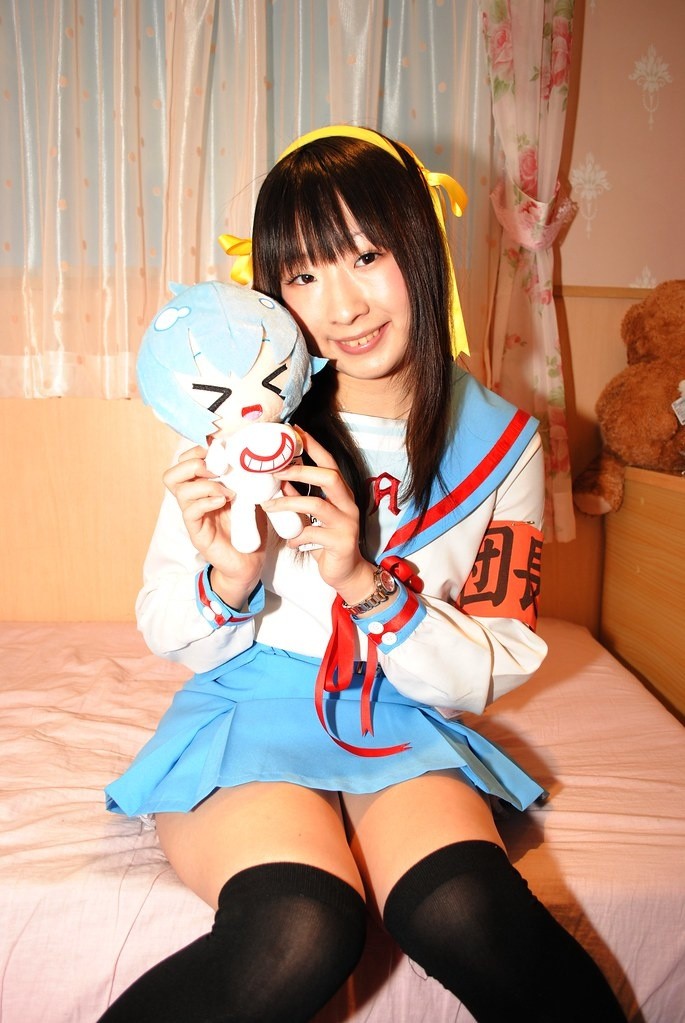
[97,127,627,1023]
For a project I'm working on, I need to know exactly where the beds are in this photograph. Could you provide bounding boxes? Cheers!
[0,618,685,1023]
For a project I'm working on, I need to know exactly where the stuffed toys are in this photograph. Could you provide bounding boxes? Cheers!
[573,280,685,516]
[136,281,309,552]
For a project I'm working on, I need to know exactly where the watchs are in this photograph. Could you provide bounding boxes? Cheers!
[341,565,395,615]
